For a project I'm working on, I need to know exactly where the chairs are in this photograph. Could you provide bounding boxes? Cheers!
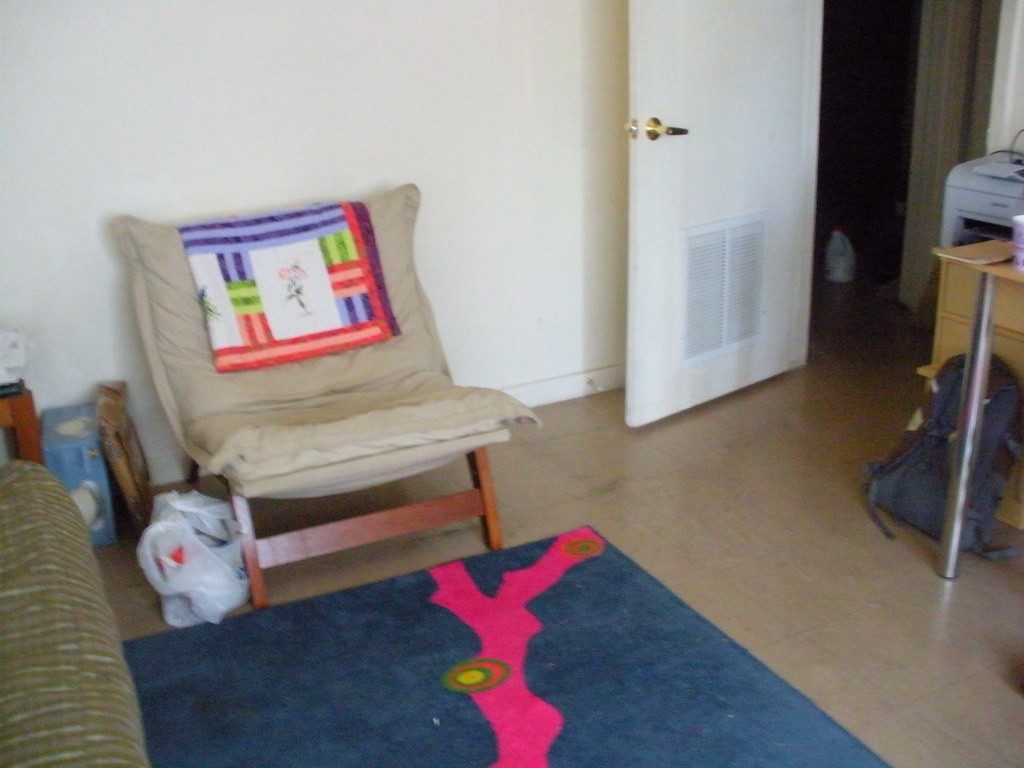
[114,183,541,606]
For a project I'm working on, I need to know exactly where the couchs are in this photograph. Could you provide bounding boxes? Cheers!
[0,459,151,768]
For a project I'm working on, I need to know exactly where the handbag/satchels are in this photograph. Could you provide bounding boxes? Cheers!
[136,491,249,628]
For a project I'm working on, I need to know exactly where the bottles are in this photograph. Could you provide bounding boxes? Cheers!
[822,225,857,282]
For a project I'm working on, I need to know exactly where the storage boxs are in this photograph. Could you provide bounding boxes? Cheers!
[41,402,116,546]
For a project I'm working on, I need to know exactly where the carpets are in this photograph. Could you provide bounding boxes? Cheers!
[131,525,893,768]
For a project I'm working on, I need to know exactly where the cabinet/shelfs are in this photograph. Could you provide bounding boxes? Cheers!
[915,257,1024,532]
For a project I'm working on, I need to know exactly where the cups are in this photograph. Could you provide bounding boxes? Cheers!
[1011,214,1024,272]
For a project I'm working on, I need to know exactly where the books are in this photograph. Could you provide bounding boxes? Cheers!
[937,240,1015,265]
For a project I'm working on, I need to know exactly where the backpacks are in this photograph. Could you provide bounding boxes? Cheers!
[868,352,1020,559]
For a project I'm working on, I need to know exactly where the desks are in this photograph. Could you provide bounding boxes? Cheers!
[0,378,44,464]
[931,240,1024,582]
